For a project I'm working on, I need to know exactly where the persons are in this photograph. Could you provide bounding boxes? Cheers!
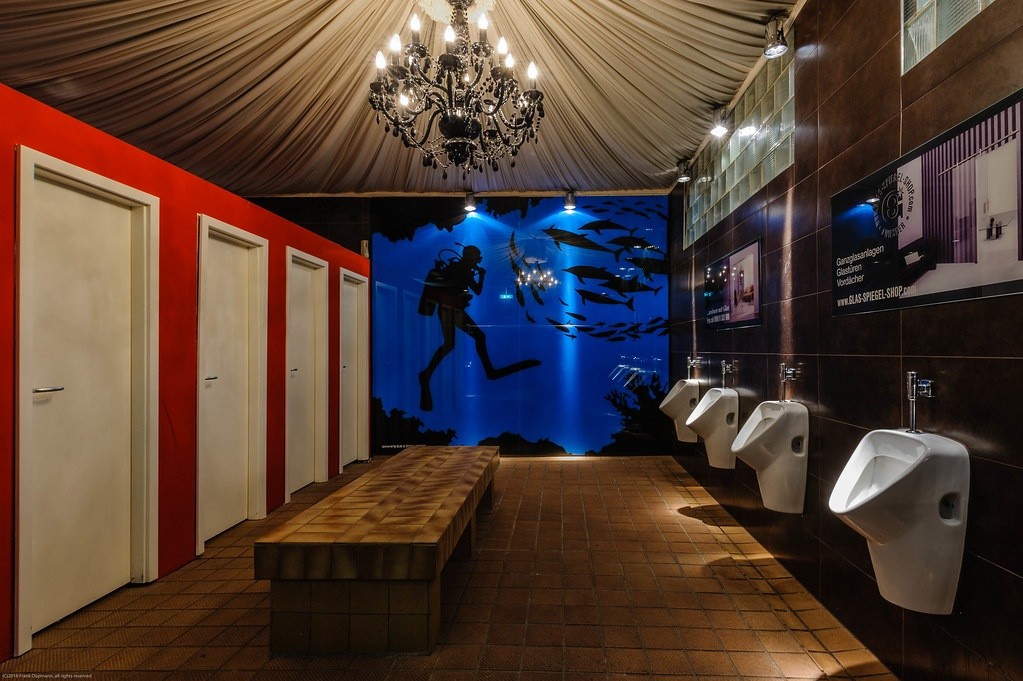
[417,245,541,412]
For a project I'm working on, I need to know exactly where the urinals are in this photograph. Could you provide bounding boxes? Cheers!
[658,378,699,443]
[684,387,739,470]
[728,398,811,514]
[826,426,972,616]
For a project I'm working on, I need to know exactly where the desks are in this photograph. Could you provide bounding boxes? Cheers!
[251,443,506,659]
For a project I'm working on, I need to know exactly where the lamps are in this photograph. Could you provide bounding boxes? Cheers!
[676,159,691,184]
[563,193,576,209]
[463,191,475,211]
[709,107,728,140]
[763,10,790,62]
[369,0,545,181]
